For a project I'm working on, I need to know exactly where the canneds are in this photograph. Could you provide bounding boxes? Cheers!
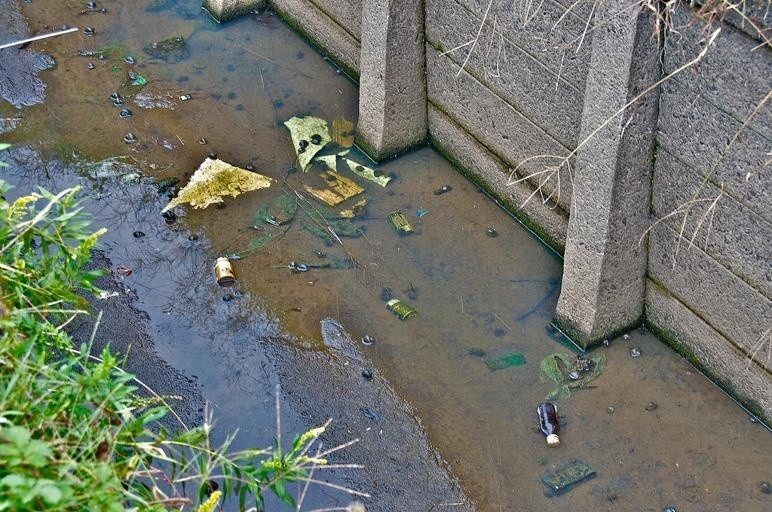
[385,298,418,321]
[387,209,414,235]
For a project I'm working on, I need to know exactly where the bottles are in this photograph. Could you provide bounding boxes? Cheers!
[536,402,562,448]
[214,256,234,283]
